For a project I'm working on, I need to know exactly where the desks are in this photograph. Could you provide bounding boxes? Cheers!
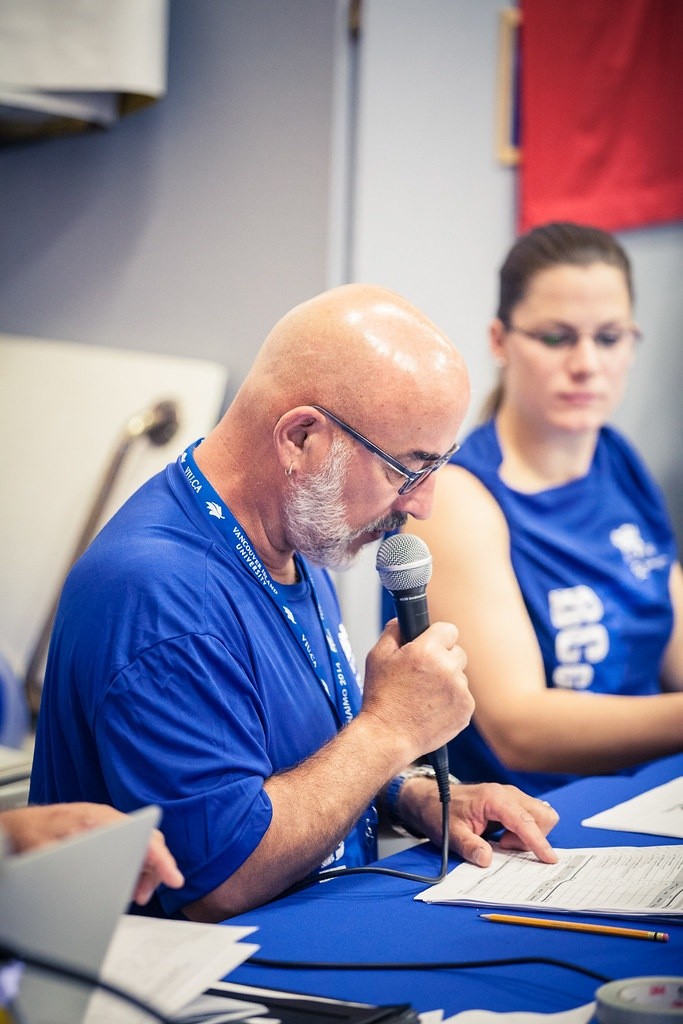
[216,752,683,1024]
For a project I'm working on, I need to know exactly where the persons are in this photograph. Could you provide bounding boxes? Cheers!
[0,803,185,904]
[379,220,683,795]
[28,284,559,927]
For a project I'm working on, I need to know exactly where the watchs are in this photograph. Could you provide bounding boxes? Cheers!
[384,764,463,841]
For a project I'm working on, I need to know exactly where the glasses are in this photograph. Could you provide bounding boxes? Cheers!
[313,405,460,496]
[512,324,643,348]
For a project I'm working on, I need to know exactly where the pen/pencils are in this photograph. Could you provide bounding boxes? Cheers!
[476,911,670,944]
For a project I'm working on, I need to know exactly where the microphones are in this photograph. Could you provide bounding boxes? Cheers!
[376,534,450,804]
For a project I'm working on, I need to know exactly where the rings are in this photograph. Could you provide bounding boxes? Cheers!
[542,800,551,808]
